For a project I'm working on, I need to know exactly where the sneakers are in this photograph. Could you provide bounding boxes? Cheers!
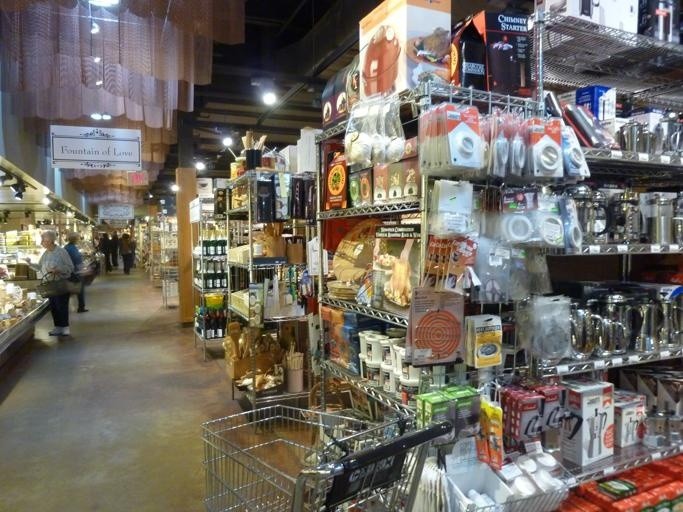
[48,326,70,336]
[76,308,89,313]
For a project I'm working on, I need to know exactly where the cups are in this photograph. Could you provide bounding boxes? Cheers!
[567,113,683,361]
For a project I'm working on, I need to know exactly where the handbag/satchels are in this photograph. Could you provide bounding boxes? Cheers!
[37,271,70,298]
[64,276,81,294]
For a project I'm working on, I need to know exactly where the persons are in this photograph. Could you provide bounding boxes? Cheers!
[64,230,89,313]
[94,231,137,275]
[23,232,74,336]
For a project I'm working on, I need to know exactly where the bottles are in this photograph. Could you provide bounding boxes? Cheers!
[193,221,227,339]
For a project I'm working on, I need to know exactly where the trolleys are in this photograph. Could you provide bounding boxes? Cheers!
[200,404,453,512]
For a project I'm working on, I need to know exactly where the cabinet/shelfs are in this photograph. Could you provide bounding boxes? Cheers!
[147,13,683,512]
